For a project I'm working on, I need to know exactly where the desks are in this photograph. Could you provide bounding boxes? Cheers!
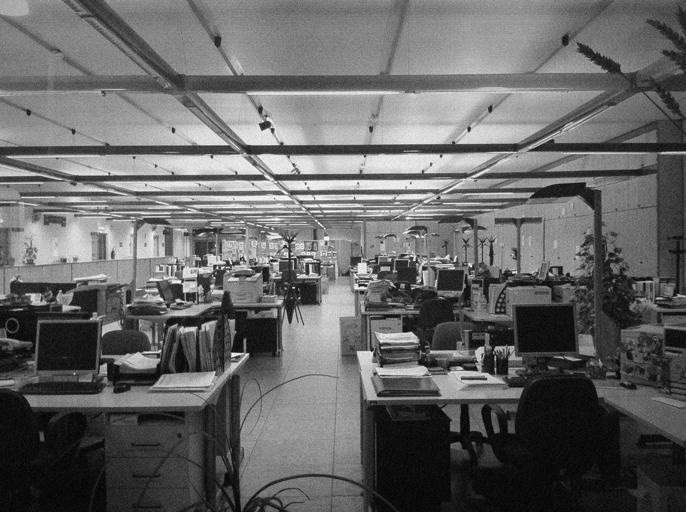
[360,291,468,348]
[605,393,685,512]
[1,350,249,511]
[359,357,666,512]
[127,288,283,358]
[356,346,599,512]
[148,254,271,288]
[355,280,421,318]
[271,272,321,305]
[6,376,228,512]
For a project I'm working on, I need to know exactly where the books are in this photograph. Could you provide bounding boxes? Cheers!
[371,361,442,397]
[162,319,236,374]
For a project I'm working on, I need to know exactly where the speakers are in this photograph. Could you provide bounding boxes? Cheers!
[5,306,27,340]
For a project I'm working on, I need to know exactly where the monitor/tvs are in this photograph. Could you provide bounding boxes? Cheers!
[513,304,579,375]
[394,259,409,270]
[378,256,387,265]
[157,280,175,303]
[539,261,550,281]
[33,319,102,381]
[436,268,464,297]
[279,260,294,272]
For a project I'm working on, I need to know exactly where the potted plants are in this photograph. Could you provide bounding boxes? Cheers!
[567,223,642,378]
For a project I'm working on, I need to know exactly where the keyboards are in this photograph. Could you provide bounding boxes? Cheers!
[19,381,106,394]
[502,373,585,387]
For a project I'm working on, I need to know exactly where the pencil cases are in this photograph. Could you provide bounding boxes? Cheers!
[370,374,441,397]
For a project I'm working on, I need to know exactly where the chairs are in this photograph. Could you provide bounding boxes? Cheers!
[378,271,394,280]
[432,321,491,461]
[372,265,379,274]
[418,299,455,331]
[481,376,599,512]
[415,291,438,303]
[394,281,409,289]
[101,330,150,355]
[0,385,86,512]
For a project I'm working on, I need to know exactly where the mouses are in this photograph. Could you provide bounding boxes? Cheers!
[114,383,131,393]
[620,380,637,390]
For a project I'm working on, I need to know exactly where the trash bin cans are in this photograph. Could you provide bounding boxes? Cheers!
[338,316,362,357]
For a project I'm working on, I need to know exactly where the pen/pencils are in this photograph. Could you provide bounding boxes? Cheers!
[482,343,515,359]
